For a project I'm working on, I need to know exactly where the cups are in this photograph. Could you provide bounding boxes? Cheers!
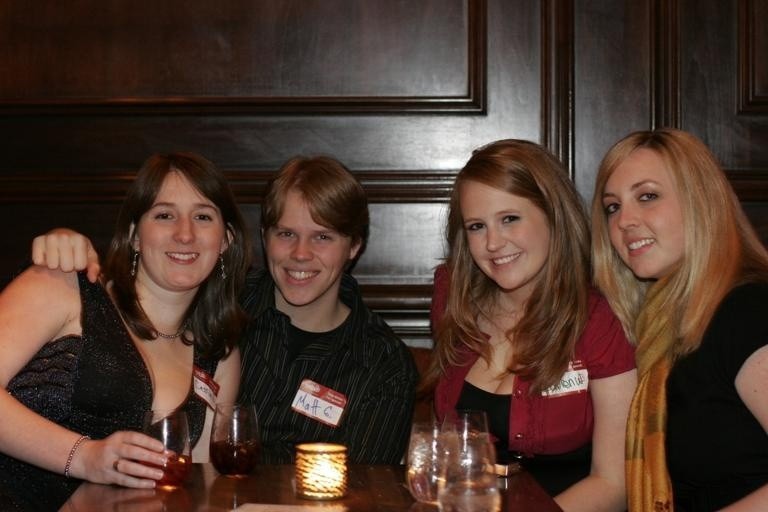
[407,410,502,512]
[206,403,261,477]
[294,444,350,500]
[142,410,196,492]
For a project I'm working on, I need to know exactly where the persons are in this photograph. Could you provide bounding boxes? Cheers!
[29,153,418,466]
[590,126,768,511]
[428,138,639,511]
[0,148,254,510]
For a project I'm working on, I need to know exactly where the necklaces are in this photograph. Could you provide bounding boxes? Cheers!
[132,289,189,339]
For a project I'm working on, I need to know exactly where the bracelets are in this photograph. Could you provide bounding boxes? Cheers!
[63,433,93,479]
